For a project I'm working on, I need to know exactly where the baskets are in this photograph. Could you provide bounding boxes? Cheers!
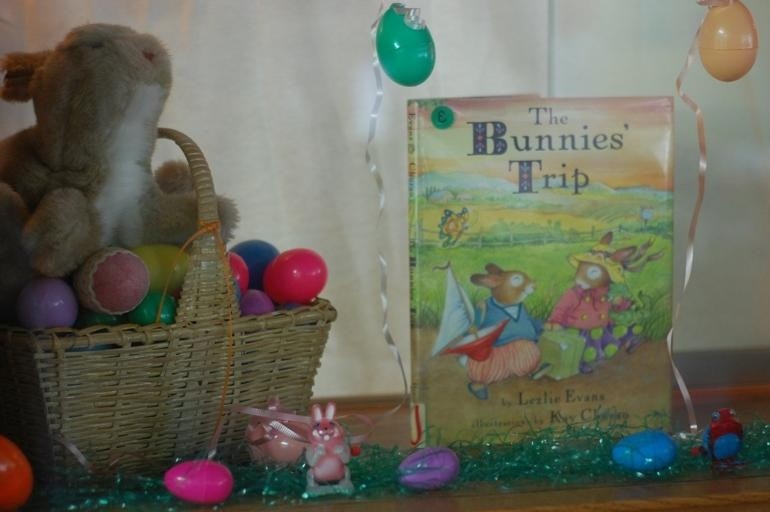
[0,126,340,505]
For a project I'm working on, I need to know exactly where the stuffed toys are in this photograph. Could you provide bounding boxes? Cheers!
[691,407,748,470]
[0,20,239,315]
[304,401,355,499]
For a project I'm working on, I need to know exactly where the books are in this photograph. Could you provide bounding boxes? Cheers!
[408,96,676,447]
